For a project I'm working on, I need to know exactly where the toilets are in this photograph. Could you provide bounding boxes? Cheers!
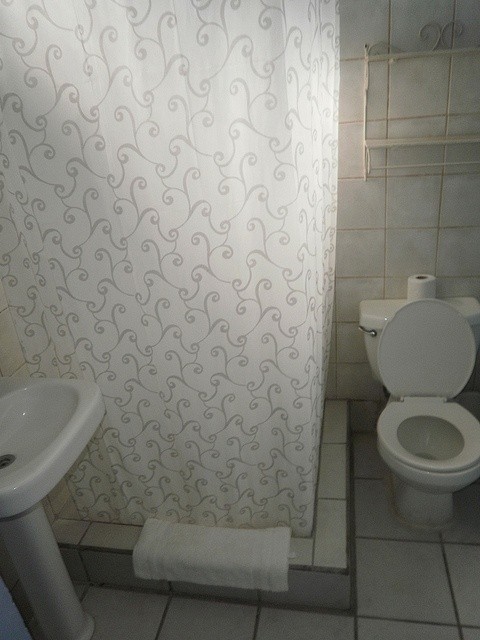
[358,297,479,527]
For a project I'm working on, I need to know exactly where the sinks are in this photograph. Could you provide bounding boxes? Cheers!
[0,377,114,519]
